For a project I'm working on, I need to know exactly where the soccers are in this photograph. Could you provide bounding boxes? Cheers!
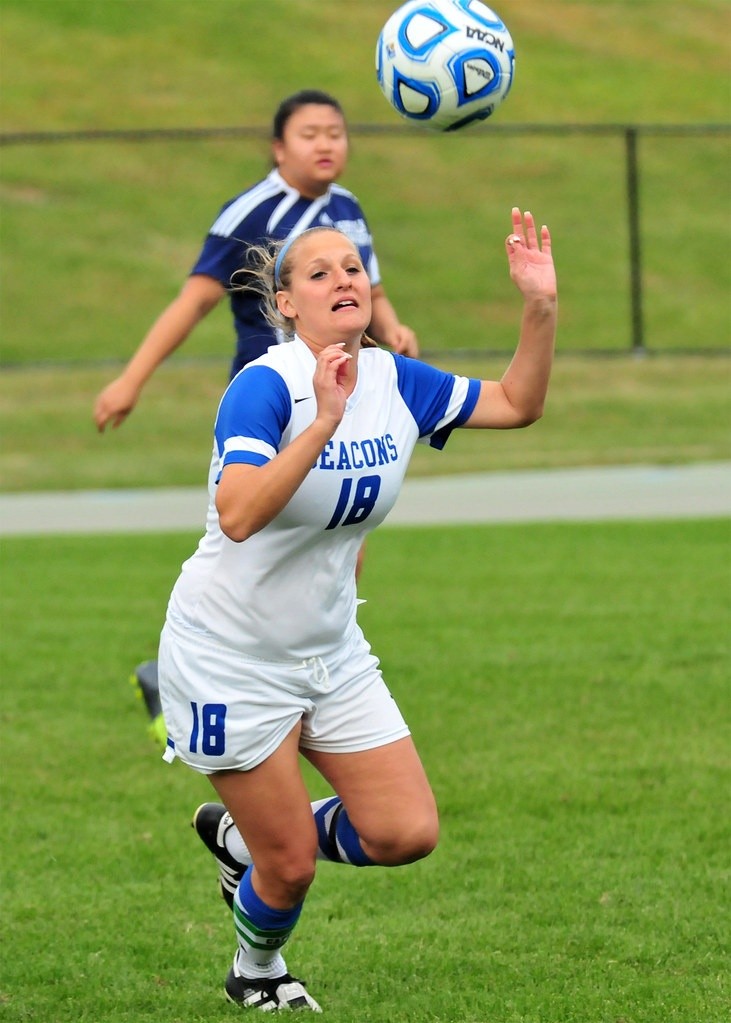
[372,0,517,134]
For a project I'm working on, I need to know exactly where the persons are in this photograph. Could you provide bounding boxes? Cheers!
[157,206,558,1008]
[90,93,419,762]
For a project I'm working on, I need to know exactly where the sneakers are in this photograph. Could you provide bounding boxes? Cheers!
[131,659,169,753]
[190,804,249,912]
[223,948,323,1013]
